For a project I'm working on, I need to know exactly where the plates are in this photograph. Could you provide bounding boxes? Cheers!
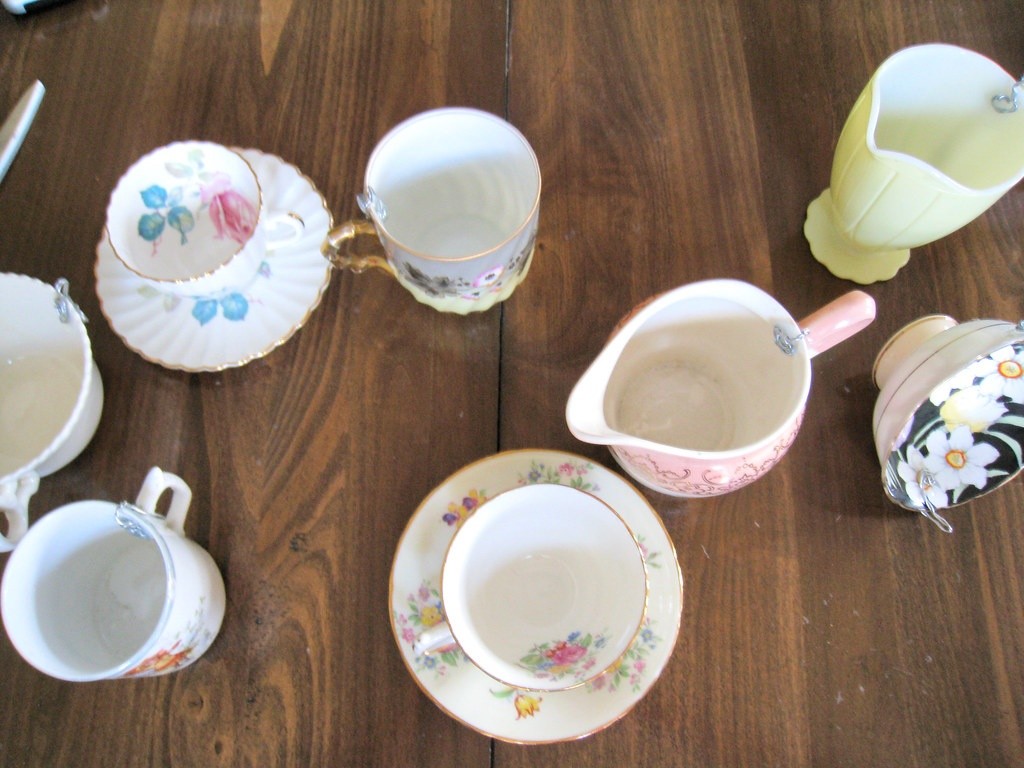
[385,448,685,743]
[95,147,340,375]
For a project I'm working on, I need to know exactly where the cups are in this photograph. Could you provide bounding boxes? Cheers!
[412,481,652,694]
[0,465,225,682]
[103,141,304,293]
[321,105,541,313]
[871,313,1024,532]
[0,271,104,558]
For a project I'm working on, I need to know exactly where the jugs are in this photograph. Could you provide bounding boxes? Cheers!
[805,43,1024,286]
[565,279,882,501]
